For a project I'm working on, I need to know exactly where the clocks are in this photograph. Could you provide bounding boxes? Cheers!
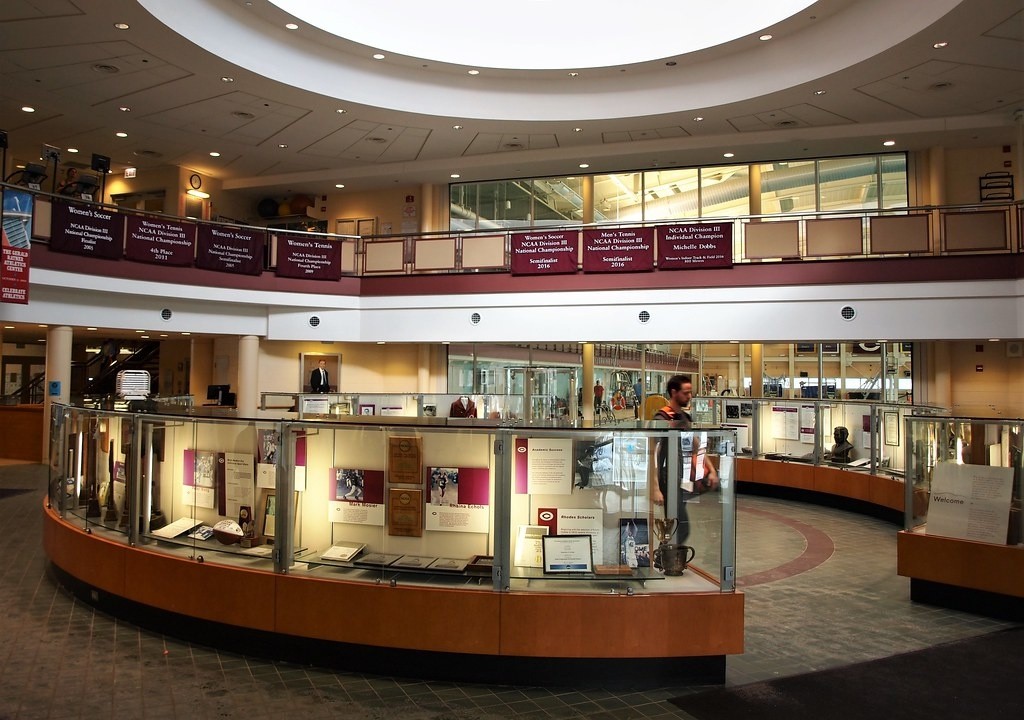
[190,174,202,189]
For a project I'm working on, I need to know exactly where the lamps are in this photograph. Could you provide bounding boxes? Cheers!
[905,371,911,375]
[800,372,808,376]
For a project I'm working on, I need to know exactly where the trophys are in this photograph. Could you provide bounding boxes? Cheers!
[653,517,680,568]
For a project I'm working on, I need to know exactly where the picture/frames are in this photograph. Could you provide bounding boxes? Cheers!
[256,488,296,544]
[884,409,900,446]
[822,343,839,354]
[543,534,594,574]
[795,343,817,353]
[359,405,376,415]
[299,353,342,394]
[900,343,911,353]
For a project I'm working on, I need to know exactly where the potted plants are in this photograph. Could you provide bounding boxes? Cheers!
[770,392,777,397]
[828,393,834,399]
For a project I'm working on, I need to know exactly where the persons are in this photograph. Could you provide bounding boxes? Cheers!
[594,380,605,415]
[431,468,458,506]
[574,446,595,489]
[336,470,364,500]
[450,396,476,417]
[262,431,278,463]
[59,168,77,196]
[610,386,627,411]
[647,374,719,550]
[622,518,650,567]
[633,378,641,419]
[705,373,712,396]
[310,360,330,392]
[831,426,854,457]
[195,452,214,483]
[548,387,582,418]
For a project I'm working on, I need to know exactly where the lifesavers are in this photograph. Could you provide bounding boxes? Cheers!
[610,395,625,410]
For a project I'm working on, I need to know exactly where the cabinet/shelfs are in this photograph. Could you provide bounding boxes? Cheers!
[979,171,1015,202]
